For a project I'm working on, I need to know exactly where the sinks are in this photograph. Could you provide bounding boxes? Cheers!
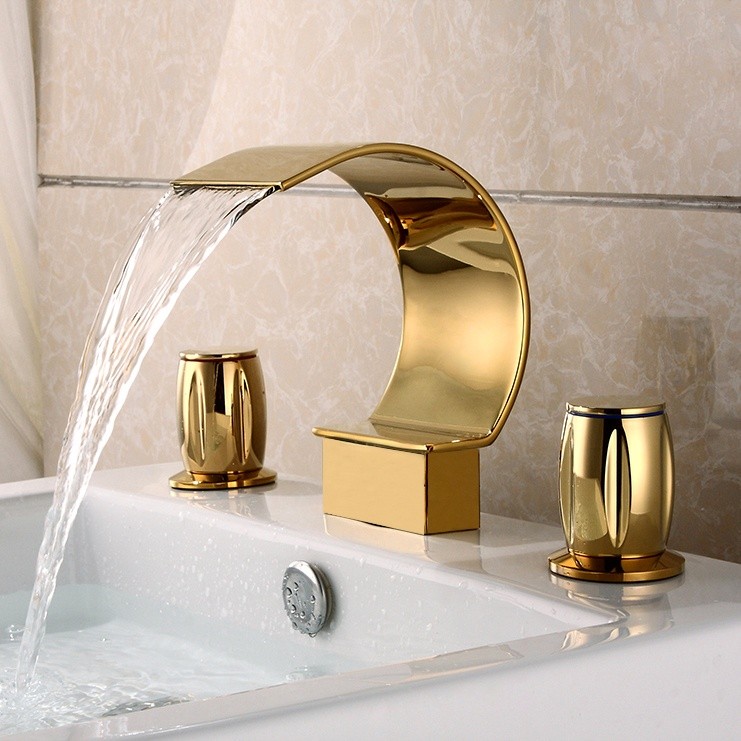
[0,471,633,739]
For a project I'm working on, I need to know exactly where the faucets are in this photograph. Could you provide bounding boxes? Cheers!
[172,136,535,542]
